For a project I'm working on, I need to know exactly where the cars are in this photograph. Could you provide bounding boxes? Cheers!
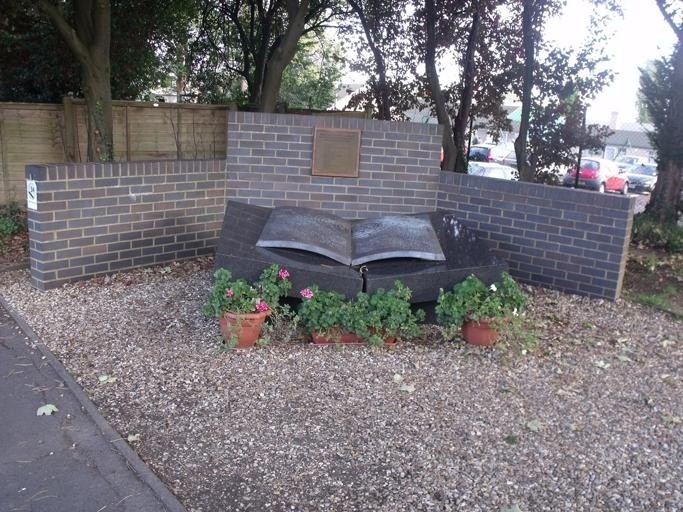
[467,142,659,195]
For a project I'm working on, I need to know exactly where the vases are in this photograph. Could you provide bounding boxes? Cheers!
[460,312,510,347]
[216,311,271,349]
[306,327,398,345]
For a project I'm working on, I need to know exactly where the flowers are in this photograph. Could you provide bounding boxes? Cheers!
[436,273,519,318]
[298,285,407,329]
[209,264,291,314]
